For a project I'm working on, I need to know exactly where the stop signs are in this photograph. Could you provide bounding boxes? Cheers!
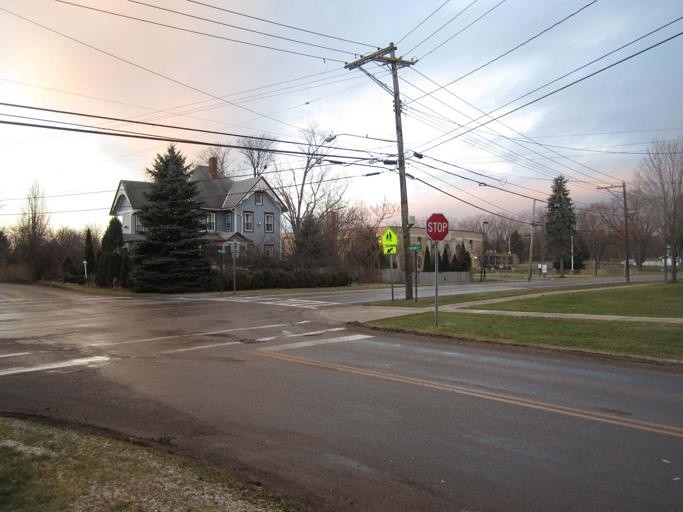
[425,213,449,242]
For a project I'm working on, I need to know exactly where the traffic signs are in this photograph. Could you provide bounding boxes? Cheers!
[216,249,228,255]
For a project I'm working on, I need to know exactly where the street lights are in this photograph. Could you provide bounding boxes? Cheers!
[596,184,631,282]
[481,221,488,257]
[325,42,413,300]
[83,260,89,282]
